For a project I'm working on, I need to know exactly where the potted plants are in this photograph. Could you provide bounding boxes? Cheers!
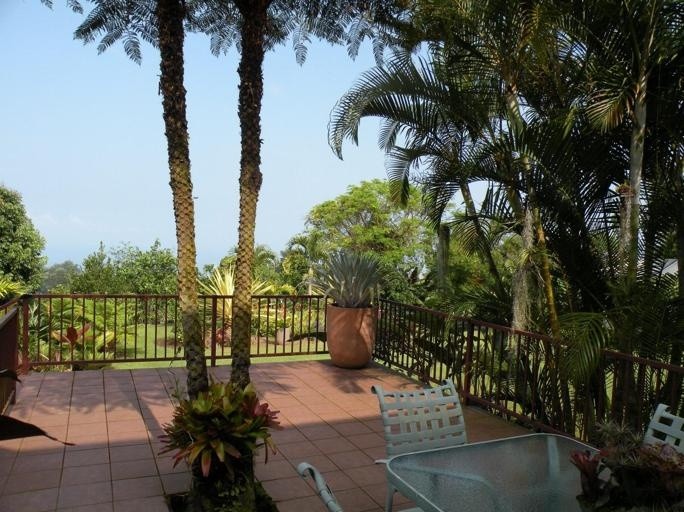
[306,247,392,372]
